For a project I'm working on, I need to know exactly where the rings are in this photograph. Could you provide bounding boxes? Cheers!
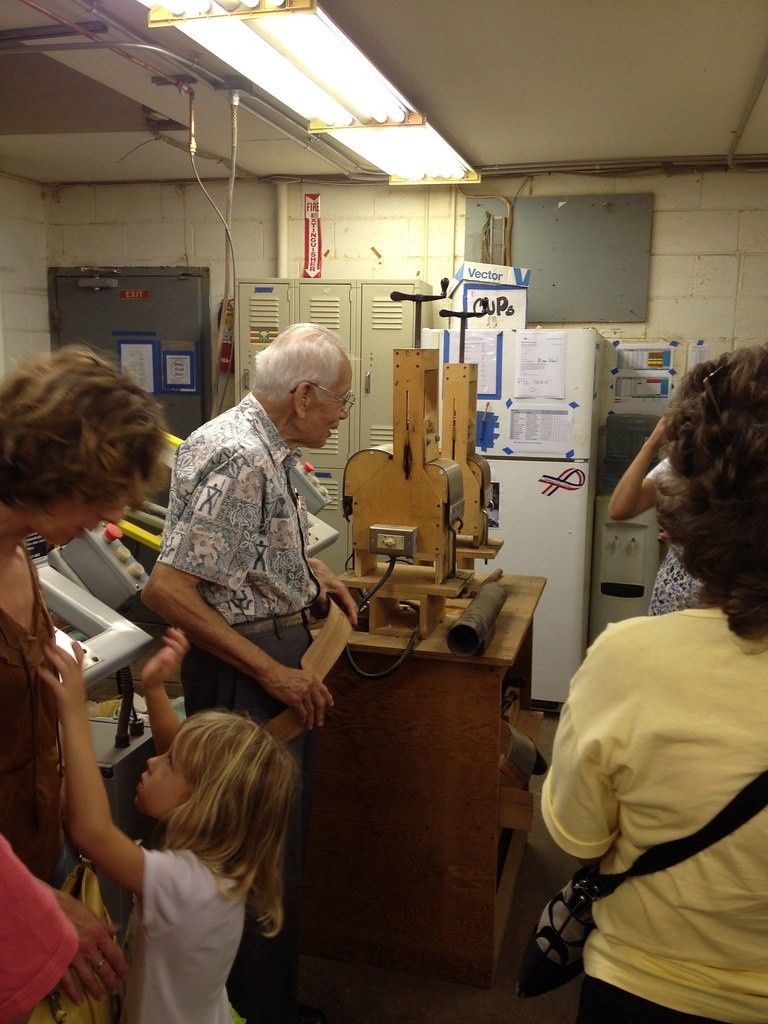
[95,959,105,971]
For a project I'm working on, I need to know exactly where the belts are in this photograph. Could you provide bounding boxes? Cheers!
[232,609,310,640]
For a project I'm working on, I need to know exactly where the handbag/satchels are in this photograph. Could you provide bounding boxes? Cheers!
[27,862,121,1024]
[517,861,599,996]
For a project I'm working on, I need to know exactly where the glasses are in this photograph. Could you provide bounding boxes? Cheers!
[290,380,356,412]
[702,363,731,419]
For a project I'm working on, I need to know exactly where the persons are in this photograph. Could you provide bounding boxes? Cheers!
[541,346,768,1024]
[142,322,359,1024]
[38,629,296,1024]
[610,412,706,617]
[1,347,168,1024]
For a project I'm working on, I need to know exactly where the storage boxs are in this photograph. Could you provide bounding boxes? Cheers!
[446,261,532,329]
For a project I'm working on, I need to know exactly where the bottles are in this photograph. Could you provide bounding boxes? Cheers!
[603,412,665,492]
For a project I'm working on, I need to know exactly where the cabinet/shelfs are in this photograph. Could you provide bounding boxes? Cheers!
[233,276,435,578]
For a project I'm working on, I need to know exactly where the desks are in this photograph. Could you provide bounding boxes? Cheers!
[300,561,547,991]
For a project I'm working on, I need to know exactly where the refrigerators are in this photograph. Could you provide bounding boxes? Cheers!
[421,325,618,714]
